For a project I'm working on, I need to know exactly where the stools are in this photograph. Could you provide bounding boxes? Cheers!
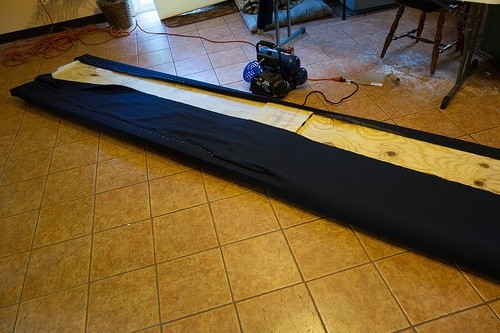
[380,0,464,75]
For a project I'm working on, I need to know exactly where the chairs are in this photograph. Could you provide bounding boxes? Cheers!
[439,0,500,109]
